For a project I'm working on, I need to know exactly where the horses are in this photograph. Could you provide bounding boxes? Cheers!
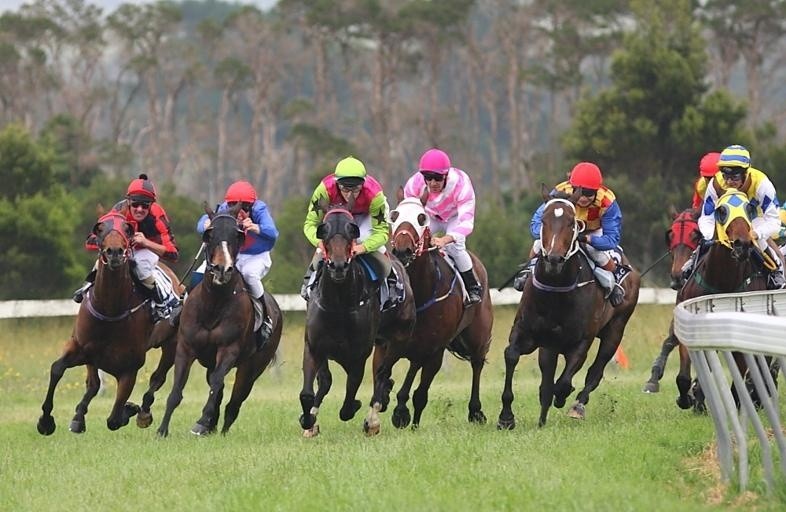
[156,198,283,438]
[642,199,704,393]
[371,185,494,432]
[298,191,418,438]
[36,201,190,436]
[496,182,642,431]
[676,172,786,417]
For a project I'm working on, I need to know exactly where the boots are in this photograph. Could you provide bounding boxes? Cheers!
[600,261,624,307]
[74,267,97,303]
[256,295,273,352]
[141,281,173,319]
[461,267,481,303]
[763,245,785,290]
[383,266,401,311]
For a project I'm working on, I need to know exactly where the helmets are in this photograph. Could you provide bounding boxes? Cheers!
[225,181,256,203]
[570,162,602,189]
[420,149,450,175]
[699,144,751,177]
[335,157,366,178]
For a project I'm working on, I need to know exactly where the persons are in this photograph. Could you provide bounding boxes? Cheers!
[512,162,627,307]
[73,173,179,326]
[171,180,279,352]
[680,143,784,292]
[406,147,483,308]
[690,151,727,210]
[300,157,404,311]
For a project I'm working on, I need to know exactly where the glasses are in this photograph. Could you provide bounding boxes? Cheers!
[132,202,149,209]
[573,188,596,196]
[342,188,361,192]
[424,174,443,180]
[230,202,250,211]
[723,172,742,180]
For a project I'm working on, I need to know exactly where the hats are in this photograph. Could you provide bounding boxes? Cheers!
[126,175,156,200]
